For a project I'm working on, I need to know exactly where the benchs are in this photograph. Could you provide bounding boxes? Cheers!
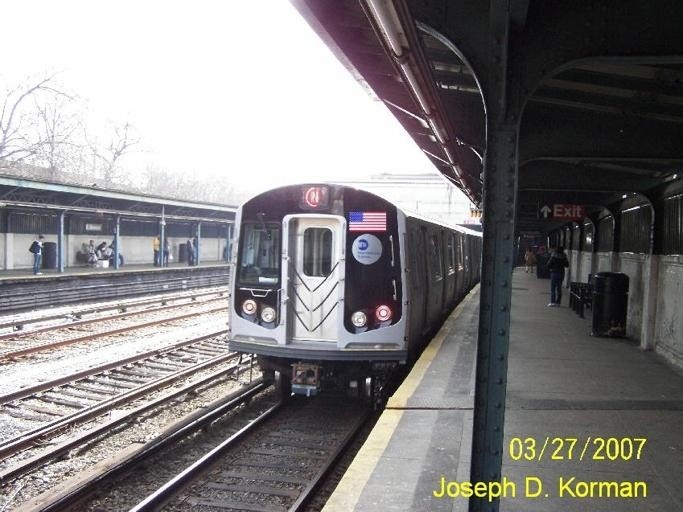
[569,274,597,318]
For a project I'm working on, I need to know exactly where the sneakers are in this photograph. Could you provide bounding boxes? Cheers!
[547,303,555,307]
[556,304,559,307]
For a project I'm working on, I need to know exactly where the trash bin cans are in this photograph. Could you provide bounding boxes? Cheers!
[179,243,189,263]
[42,242,56,270]
[592,270,630,337]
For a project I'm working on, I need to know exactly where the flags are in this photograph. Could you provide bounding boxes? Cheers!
[349,211,387,232]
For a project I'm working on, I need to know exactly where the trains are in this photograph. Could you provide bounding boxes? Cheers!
[225,180,484,406]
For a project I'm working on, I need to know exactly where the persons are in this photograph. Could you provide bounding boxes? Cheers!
[187,237,195,266]
[33,237,44,275]
[524,249,536,273]
[81,240,123,267]
[153,233,160,267]
[163,237,170,267]
[547,246,569,307]
[193,236,198,265]
